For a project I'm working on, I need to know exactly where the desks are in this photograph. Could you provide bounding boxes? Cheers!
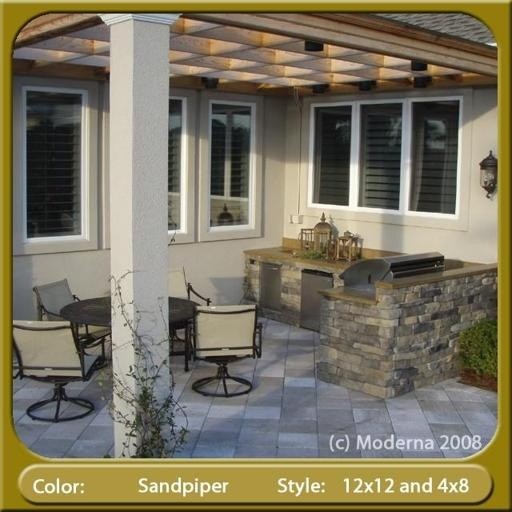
[59,297,201,372]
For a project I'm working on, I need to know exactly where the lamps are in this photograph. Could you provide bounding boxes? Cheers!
[479,150,498,198]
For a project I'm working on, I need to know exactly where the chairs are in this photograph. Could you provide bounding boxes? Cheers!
[12,277,111,422]
[168,267,263,397]
